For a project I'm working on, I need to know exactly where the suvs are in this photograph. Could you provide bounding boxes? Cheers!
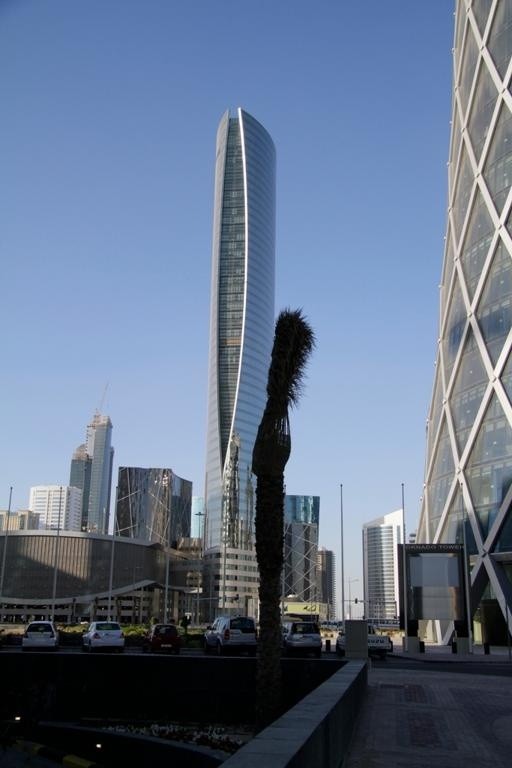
[280,622,322,658]
[204,615,257,656]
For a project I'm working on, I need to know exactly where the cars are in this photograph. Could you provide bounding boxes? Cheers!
[22,620,60,648]
[143,624,180,654]
[82,621,125,653]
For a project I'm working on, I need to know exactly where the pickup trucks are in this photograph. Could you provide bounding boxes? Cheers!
[335,625,393,660]
